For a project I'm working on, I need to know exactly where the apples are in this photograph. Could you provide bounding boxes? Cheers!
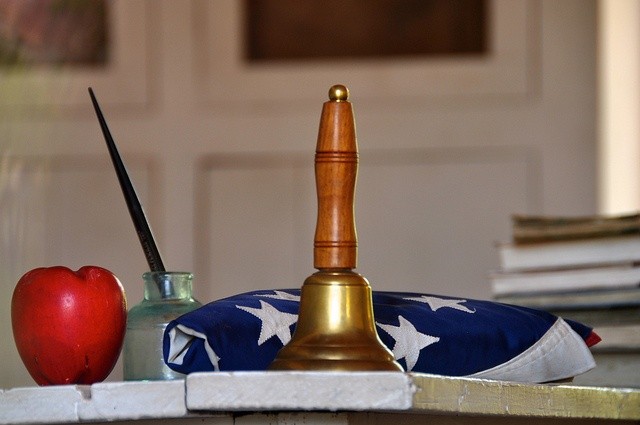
[11,262,127,386]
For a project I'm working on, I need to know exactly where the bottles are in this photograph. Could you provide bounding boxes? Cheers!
[123,271,203,382]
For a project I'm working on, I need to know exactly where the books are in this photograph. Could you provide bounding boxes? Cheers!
[490,213,640,355]
